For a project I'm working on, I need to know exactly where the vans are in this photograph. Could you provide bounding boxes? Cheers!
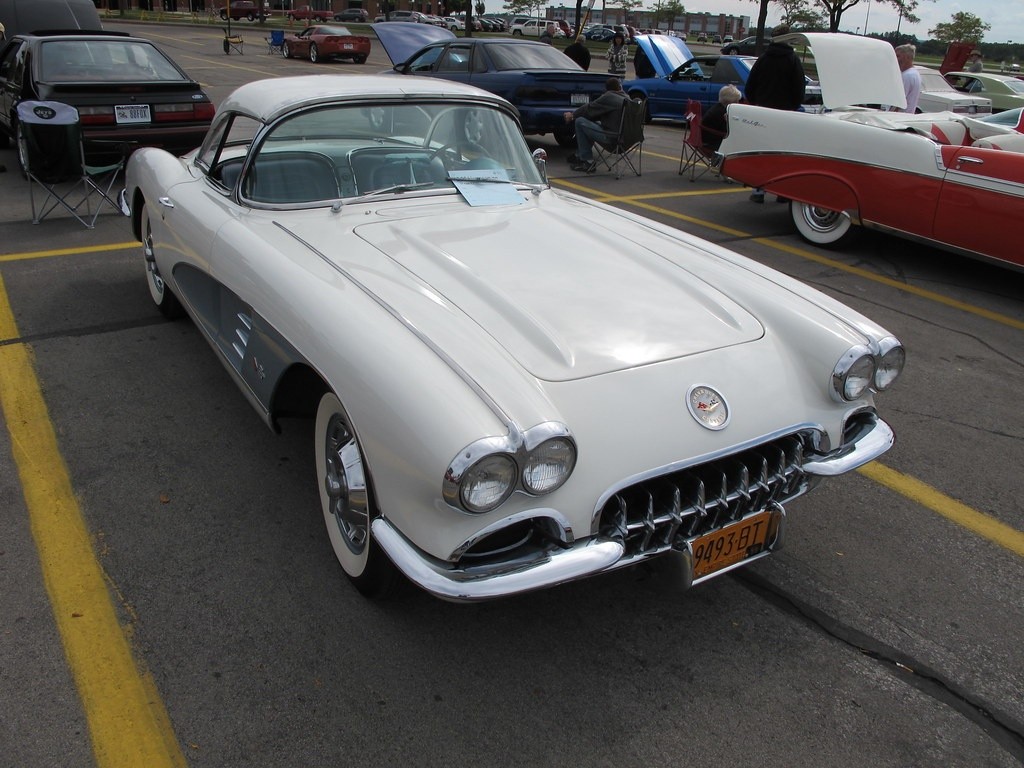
[583,24,630,43]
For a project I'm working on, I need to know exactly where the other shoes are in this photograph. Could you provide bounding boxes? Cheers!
[749,194,764,203]
[574,161,596,173]
[775,196,790,203]
[567,154,581,165]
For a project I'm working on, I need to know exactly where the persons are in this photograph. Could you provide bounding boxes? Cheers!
[563,77,632,172]
[885,44,920,114]
[605,32,628,79]
[563,35,591,72]
[538,24,555,46]
[702,84,742,150]
[744,25,805,202]
[963,50,983,91]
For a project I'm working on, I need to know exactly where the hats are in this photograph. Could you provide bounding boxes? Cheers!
[970,50,984,58]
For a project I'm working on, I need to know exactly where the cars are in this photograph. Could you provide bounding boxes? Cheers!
[1006,64,1020,71]
[581,28,617,41]
[371,22,624,149]
[623,34,814,124]
[723,35,733,43]
[557,30,566,39]
[0,0,216,181]
[427,14,466,31]
[460,17,508,32]
[710,32,1024,279]
[913,64,1024,114]
[281,25,372,65]
[627,27,687,42]
[118,72,907,610]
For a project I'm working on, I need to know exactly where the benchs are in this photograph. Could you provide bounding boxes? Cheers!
[896,121,969,147]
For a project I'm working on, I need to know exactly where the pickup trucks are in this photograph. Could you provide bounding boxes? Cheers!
[285,5,333,22]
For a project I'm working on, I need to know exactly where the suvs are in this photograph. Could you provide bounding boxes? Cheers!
[374,10,428,23]
[696,33,707,42]
[219,0,272,21]
[509,20,562,38]
[509,17,534,29]
[334,8,369,22]
[555,20,572,38]
[712,35,722,44]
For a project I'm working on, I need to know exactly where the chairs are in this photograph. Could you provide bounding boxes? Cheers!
[586,98,648,181]
[263,30,285,55]
[678,98,731,184]
[971,134,1024,153]
[223,27,243,55]
[346,146,448,197]
[213,152,341,202]
[17,101,137,229]
